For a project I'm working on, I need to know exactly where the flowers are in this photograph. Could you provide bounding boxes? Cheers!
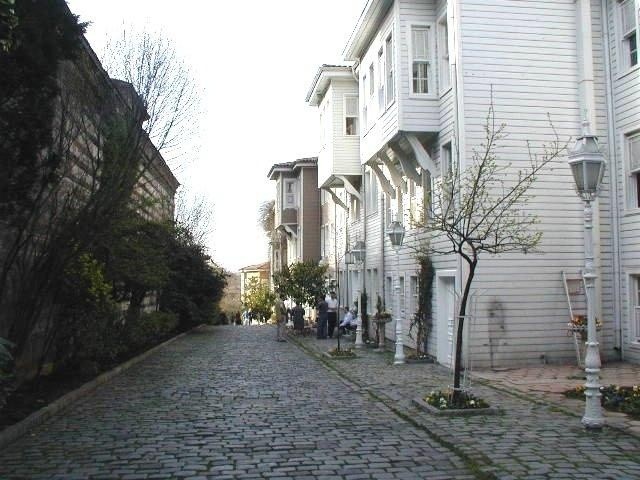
[567,315,602,329]
[370,307,393,320]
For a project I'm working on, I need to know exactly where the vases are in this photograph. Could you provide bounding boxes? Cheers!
[373,317,387,354]
[574,325,593,361]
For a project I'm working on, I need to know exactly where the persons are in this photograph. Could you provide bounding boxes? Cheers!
[228,289,357,342]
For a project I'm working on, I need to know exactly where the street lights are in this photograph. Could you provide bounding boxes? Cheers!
[567,132,607,432]
[350,241,367,348]
[386,219,405,365]
